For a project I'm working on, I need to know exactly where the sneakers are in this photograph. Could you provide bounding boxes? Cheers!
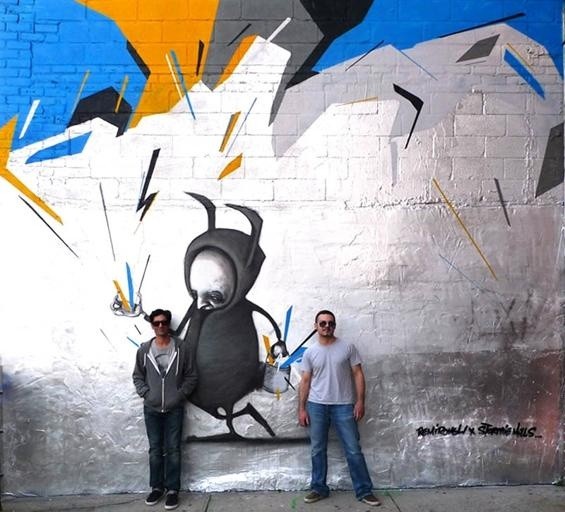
[362,495,380,506]
[145,489,165,505]
[165,493,178,510]
[304,490,323,503]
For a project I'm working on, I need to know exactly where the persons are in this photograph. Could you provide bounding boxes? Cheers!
[132,309,200,509]
[298,309,381,506]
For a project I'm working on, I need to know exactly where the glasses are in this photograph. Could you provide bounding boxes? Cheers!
[152,320,170,327]
[319,321,335,327]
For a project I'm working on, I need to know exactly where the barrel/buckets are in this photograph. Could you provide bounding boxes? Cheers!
[261,360,292,394]
